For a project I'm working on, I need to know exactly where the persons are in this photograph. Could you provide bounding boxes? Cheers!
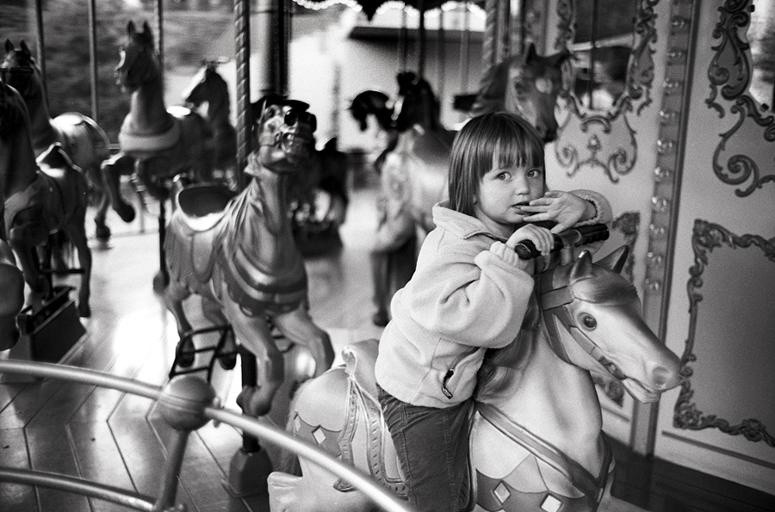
[370,109,616,509]
